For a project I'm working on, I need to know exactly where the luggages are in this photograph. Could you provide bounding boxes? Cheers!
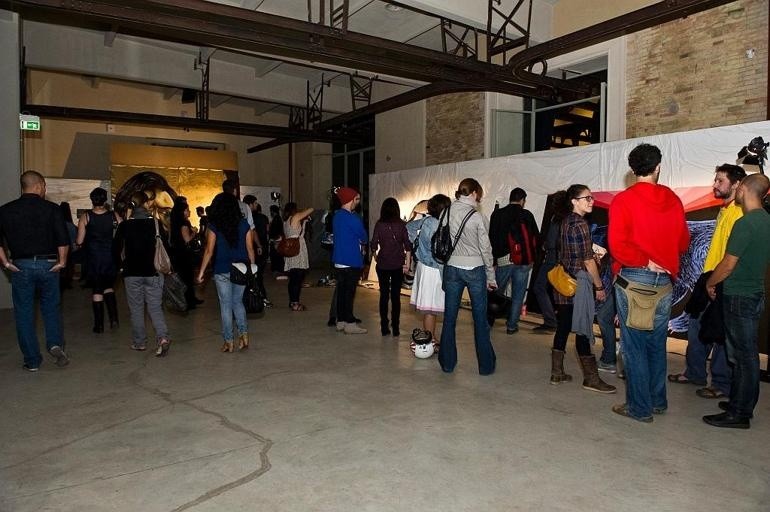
[243,273,264,314]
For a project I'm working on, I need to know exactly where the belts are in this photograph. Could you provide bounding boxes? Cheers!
[26,254,57,259]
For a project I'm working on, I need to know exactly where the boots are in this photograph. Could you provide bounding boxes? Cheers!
[550,347,572,385]
[578,353,617,394]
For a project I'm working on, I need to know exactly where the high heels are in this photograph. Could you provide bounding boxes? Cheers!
[238,333,250,351]
[220,341,236,354]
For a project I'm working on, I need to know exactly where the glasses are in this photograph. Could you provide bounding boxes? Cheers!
[574,196,593,202]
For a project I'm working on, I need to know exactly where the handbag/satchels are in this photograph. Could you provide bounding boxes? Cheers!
[80,240,106,273]
[507,222,538,265]
[154,237,172,274]
[547,263,578,297]
[184,235,202,255]
[410,332,436,359]
[229,262,255,286]
[431,224,452,262]
[277,238,300,257]
[625,282,659,331]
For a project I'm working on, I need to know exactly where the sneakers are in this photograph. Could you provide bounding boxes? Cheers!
[50,345,70,367]
[597,360,624,378]
[668,372,692,384]
[382,326,400,336]
[22,363,39,372]
[110,321,118,329]
[92,323,104,333]
[506,328,519,334]
[613,405,665,422]
[128,343,146,351]
[533,324,556,332]
[153,337,170,357]
[262,298,274,308]
[328,316,368,334]
[696,386,727,398]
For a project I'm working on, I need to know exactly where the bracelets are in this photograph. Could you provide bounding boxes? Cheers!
[5,261,10,269]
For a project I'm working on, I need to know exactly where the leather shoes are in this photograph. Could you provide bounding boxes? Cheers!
[703,411,749,429]
[718,401,754,418]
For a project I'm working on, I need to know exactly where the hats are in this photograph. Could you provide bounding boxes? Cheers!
[335,188,358,204]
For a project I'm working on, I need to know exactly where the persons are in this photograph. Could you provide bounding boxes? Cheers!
[61,180,369,356]
[0,171,71,372]
[607,145,690,423]
[370,197,411,336]
[668,165,747,398]
[703,174,770,429]
[406,178,624,393]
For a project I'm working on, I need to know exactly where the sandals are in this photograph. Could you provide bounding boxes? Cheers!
[289,301,308,311]
